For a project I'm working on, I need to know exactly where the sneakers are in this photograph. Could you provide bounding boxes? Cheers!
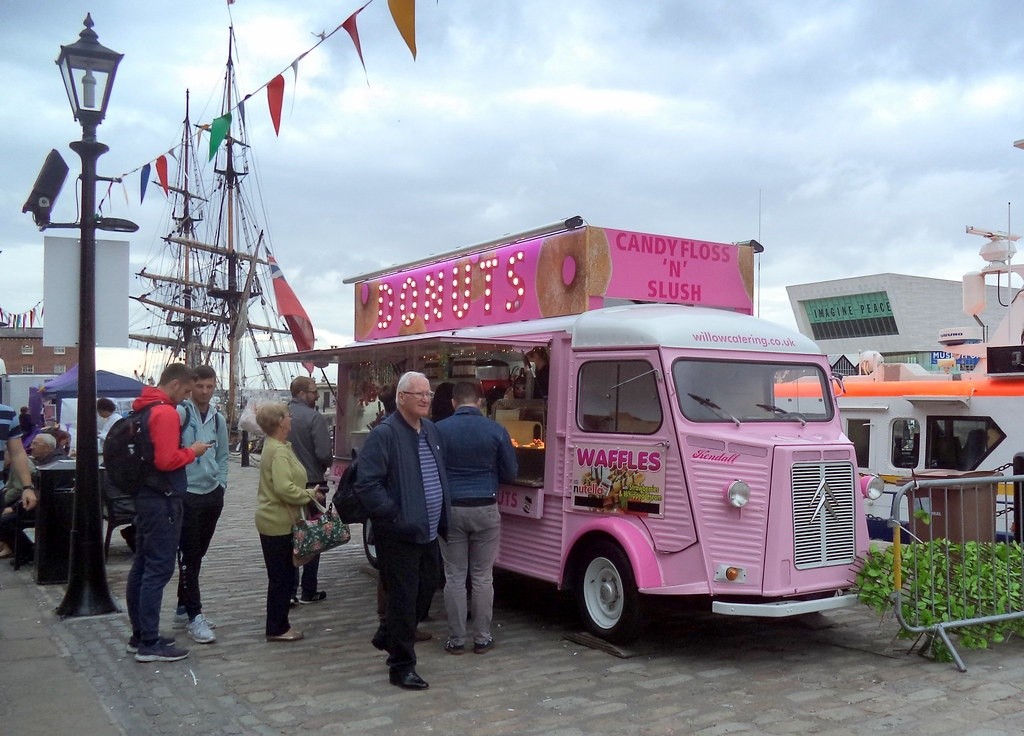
[126,633,175,652]
[135,641,190,662]
[475,636,496,654]
[172,612,216,629]
[445,638,464,655]
[186,613,216,643]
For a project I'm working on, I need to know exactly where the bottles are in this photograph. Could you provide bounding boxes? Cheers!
[452,357,476,377]
[423,360,440,379]
[512,367,535,401]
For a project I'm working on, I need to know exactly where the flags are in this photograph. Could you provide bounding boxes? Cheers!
[262,239,315,373]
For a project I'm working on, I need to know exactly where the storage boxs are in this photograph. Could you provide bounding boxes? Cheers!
[584,411,651,434]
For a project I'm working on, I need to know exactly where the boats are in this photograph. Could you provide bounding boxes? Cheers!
[773,198,1024,546]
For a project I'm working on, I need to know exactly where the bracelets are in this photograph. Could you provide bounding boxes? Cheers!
[23,486,34,489]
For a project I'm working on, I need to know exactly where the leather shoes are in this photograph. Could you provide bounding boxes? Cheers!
[371,627,390,654]
[389,669,429,689]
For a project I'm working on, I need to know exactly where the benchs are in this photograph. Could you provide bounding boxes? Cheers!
[12,467,137,572]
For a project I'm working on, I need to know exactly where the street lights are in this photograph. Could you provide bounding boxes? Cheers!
[21,10,146,613]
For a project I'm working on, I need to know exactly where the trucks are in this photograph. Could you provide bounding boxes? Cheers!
[256,214,884,648]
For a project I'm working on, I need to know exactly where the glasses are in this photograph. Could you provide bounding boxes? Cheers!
[298,389,318,396]
[402,389,435,400]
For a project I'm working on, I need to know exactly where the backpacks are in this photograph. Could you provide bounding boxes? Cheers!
[103,399,175,493]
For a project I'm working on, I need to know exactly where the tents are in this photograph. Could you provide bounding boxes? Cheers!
[43,369,148,431]
[27,362,79,447]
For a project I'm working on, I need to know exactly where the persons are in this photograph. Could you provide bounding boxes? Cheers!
[126,363,215,662]
[959,429,989,469]
[431,382,516,655]
[353,370,451,689]
[104,472,137,554]
[0,404,74,566]
[171,366,228,642]
[255,401,326,641]
[97,398,124,450]
[377,385,434,638]
[289,376,333,607]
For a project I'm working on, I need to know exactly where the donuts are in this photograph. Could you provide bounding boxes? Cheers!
[536,227,612,317]
[353,280,379,341]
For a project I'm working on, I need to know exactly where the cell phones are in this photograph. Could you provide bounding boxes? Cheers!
[203,440,216,445]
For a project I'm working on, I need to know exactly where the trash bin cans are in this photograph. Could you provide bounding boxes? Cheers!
[32,459,106,584]
[895,469,1004,599]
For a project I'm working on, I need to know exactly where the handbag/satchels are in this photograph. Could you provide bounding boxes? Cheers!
[334,422,398,525]
[291,491,350,567]
[236,398,263,432]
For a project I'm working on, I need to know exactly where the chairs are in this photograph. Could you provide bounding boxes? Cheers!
[911,433,920,456]
[938,428,989,470]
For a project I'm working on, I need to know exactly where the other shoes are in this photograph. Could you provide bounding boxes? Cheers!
[417,613,433,621]
[0,539,37,566]
[267,628,304,641]
[413,629,433,641]
[299,590,327,603]
[120,524,138,552]
[290,597,298,603]
